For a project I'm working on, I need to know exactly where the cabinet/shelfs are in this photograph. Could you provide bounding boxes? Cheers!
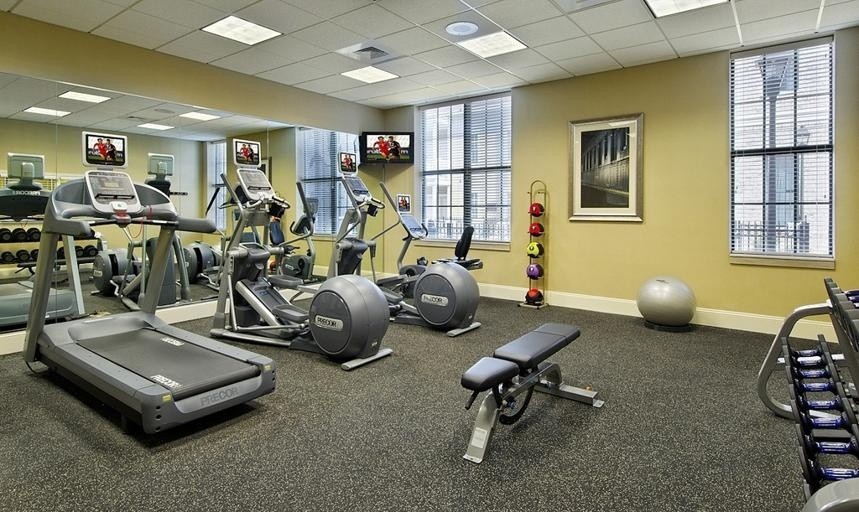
[0,221,102,274]
[756,279,859,511]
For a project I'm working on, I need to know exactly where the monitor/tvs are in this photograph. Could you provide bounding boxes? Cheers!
[233,138,262,169]
[397,194,412,213]
[361,131,414,163]
[147,153,174,181]
[7,152,45,185]
[338,152,358,176]
[302,198,319,214]
[81,131,129,169]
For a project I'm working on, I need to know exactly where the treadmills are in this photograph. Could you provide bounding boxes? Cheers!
[0,152,86,329]
[25,131,273,436]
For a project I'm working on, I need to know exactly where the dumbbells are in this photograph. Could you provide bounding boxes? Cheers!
[1,227,97,263]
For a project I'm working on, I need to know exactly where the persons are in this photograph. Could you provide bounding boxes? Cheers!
[94,139,116,161]
[373,136,400,159]
[400,198,408,209]
[344,155,353,172]
[240,144,254,162]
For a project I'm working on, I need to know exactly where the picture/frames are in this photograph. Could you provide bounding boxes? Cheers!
[567,111,645,222]
